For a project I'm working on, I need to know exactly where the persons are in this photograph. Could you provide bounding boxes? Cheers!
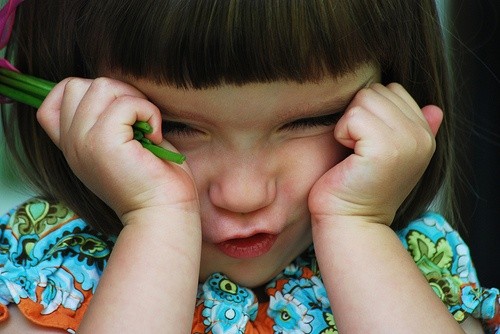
[2,0,500,334]
